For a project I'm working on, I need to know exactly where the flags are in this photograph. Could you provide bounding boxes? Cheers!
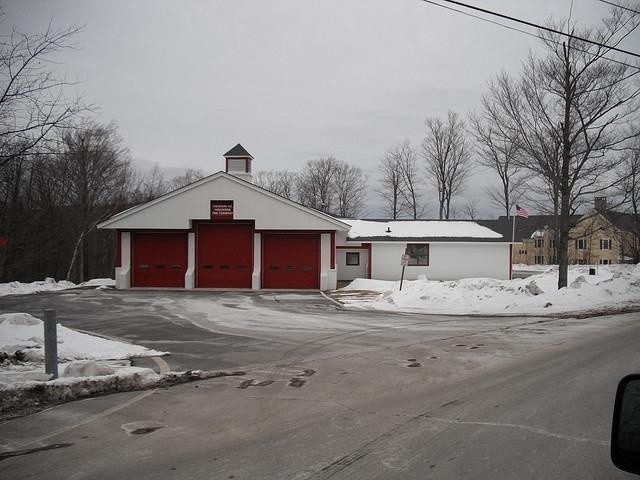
[514,204,529,219]
